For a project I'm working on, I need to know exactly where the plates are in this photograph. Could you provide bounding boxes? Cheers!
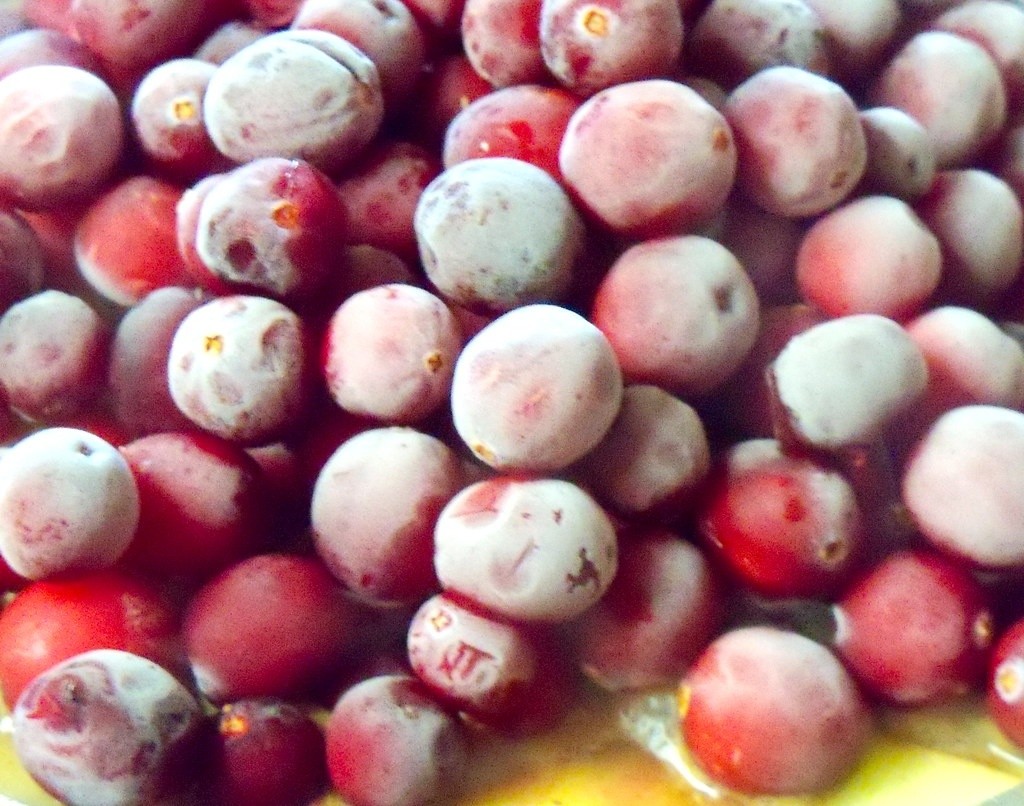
[1,675,1024,806]
